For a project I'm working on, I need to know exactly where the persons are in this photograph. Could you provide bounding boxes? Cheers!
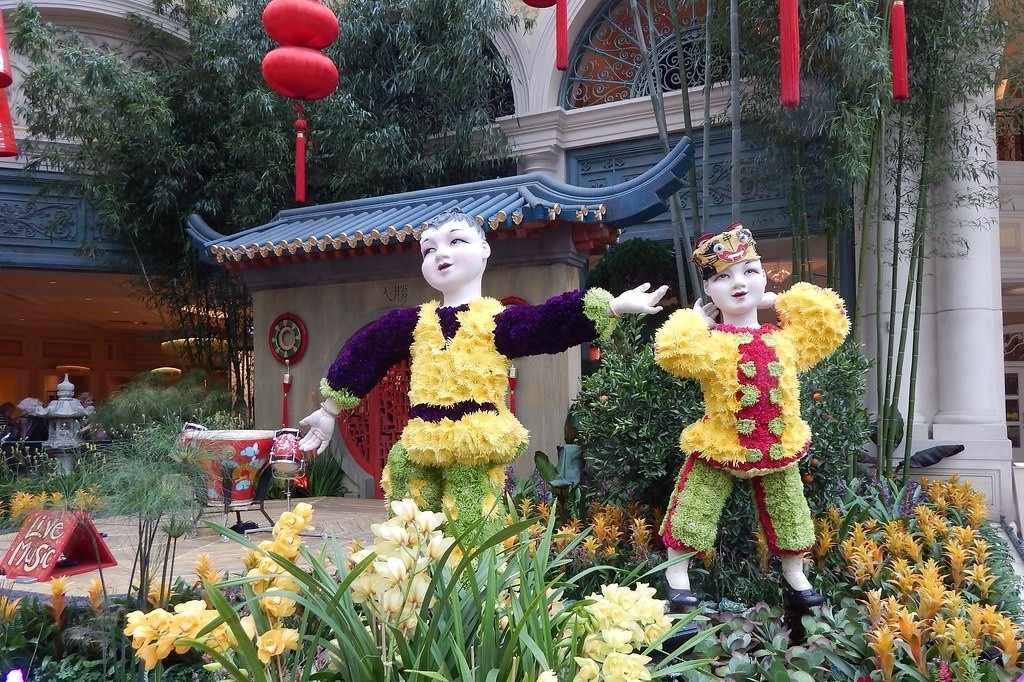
[0,402,17,447]
[299,211,668,556]
[79,391,93,408]
[651,221,850,608]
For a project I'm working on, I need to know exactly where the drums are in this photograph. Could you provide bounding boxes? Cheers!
[271,426,305,474]
[183,428,277,511]
[169,422,208,465]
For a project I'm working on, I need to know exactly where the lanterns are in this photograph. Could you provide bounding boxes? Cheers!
[524,0,570,71]
[259,0,339,203]
